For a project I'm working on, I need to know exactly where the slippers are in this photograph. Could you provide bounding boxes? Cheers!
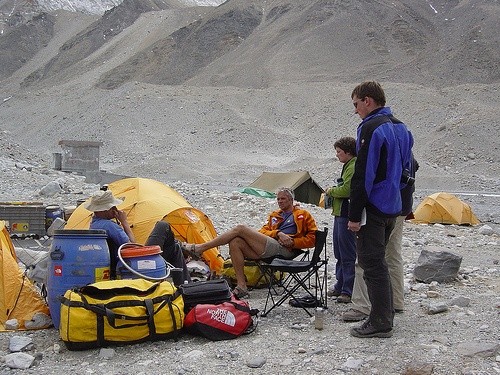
[231,286,249,297]
[180,241,202,261]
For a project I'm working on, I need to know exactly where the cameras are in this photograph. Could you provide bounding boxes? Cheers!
[400,168,416,187]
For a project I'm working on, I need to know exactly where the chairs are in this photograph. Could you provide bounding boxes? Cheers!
[249,226,330,317]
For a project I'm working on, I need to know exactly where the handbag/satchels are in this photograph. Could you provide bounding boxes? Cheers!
[57,279,260,351]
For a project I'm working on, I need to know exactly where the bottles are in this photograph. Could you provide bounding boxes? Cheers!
[314,307,324,329]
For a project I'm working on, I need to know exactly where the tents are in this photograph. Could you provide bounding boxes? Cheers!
[411,192,480,225]
[238,187,276,198]
[64,177,225,275]
[0,220,51,333]
[249,170,325,205]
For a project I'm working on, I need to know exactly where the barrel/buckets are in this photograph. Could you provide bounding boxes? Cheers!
[46,205,63,228]
[46,229,110,329]
[115,245,167,282]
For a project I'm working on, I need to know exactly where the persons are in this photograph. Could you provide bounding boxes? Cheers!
[180,189,318,299]
[342,151,419,321]
[84,189,188,284]
[325,136,358,302]
[347,81,415,338]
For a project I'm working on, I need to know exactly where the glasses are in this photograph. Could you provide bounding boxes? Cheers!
[353,96,367,108]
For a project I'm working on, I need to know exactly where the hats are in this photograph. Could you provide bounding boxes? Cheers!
[85,189,121,212]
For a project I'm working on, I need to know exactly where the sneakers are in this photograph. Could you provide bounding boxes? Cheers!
[343,307,366,320]
[327,290,339,296]
[350,318,393,338]
[337,294,351,302]
